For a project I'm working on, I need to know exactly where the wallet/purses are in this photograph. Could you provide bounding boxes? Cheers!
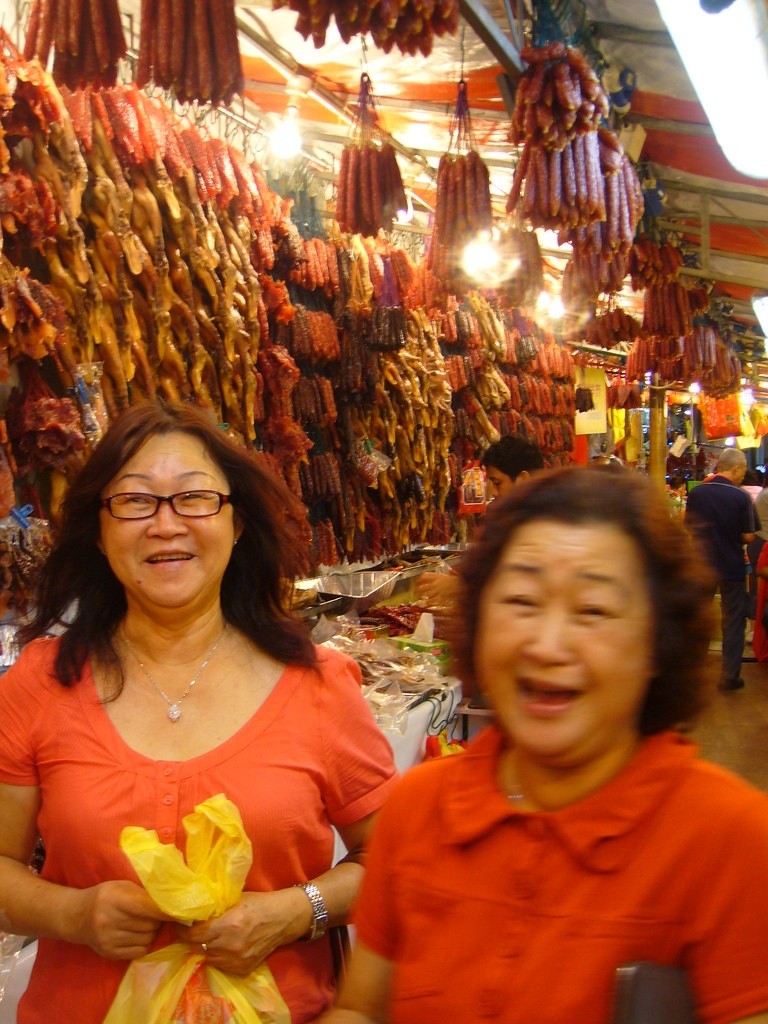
[612,963,698,1024]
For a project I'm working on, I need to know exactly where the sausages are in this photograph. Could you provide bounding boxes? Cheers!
[22,0,743,571]
[365,603,464,643]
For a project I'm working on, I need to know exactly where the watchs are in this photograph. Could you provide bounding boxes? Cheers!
[294,882,328,943]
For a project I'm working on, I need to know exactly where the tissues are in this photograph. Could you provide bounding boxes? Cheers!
[386,612,450,660]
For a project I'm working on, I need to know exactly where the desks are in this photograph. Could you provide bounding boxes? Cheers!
[330,675,461,869]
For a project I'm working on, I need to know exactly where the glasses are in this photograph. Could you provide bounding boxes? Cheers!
[100,490,236,521]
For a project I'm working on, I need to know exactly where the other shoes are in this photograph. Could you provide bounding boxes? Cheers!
[745,631,754,643]
[717,678,744,690]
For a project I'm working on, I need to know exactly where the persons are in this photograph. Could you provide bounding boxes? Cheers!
[415,435,544,607]
[683,447,768,692]
[0,399,401,1024]
[317,465,768,1024]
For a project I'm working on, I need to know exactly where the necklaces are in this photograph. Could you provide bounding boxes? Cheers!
[121,627,229,721]
[508,786,526,803]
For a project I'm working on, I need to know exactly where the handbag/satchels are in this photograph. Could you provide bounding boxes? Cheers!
[97,795,296,1024]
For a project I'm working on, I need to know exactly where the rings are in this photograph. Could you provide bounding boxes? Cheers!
[201,943,208,950]
